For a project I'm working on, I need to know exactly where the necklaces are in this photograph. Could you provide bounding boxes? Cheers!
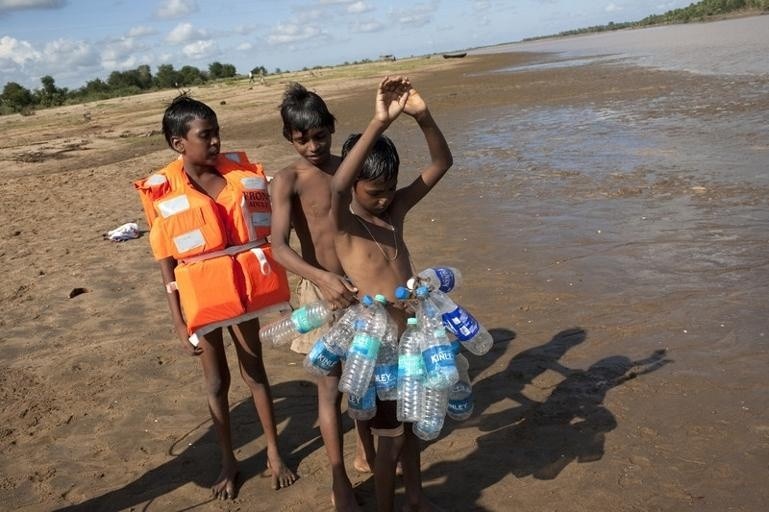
[356,212,398,262]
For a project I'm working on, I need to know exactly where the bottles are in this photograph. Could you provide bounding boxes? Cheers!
[253,267,492,441]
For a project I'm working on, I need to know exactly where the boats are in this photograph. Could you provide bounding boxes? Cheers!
[443,53,467,60]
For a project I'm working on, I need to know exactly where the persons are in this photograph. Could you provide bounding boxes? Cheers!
[144,97,300,501]
[271,83,403,512]
[331,74,453,512]
[248,70,256,84]
